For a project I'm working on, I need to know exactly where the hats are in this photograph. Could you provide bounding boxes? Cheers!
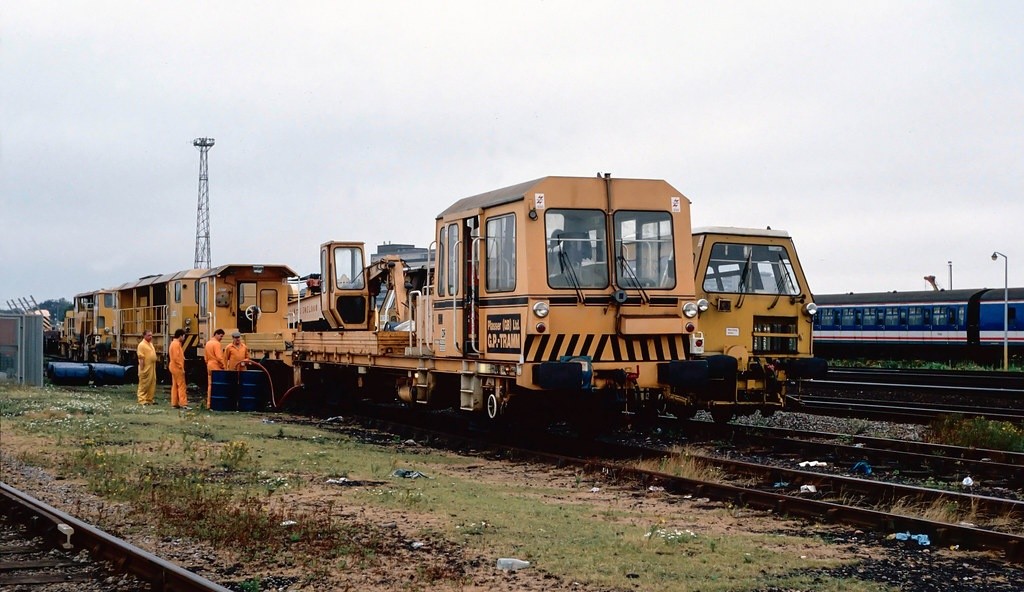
[231,332,241,338]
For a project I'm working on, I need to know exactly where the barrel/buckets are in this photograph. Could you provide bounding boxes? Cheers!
[47,360,138,385]
[237,369,266,412]
[210,369,239,412]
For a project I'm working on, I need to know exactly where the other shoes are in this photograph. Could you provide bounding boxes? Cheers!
[173,405,193,411]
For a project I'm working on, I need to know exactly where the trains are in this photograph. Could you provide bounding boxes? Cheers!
[597,227,820,426]
[802,285,1023,359]
[46,171,707,443]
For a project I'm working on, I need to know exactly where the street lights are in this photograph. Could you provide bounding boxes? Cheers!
[992,251,1009,370]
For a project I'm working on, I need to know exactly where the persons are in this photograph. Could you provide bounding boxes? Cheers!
[202,328,227,410]
[224,331,251,370]
[168,328,194,410]
[136,330,160,406]
